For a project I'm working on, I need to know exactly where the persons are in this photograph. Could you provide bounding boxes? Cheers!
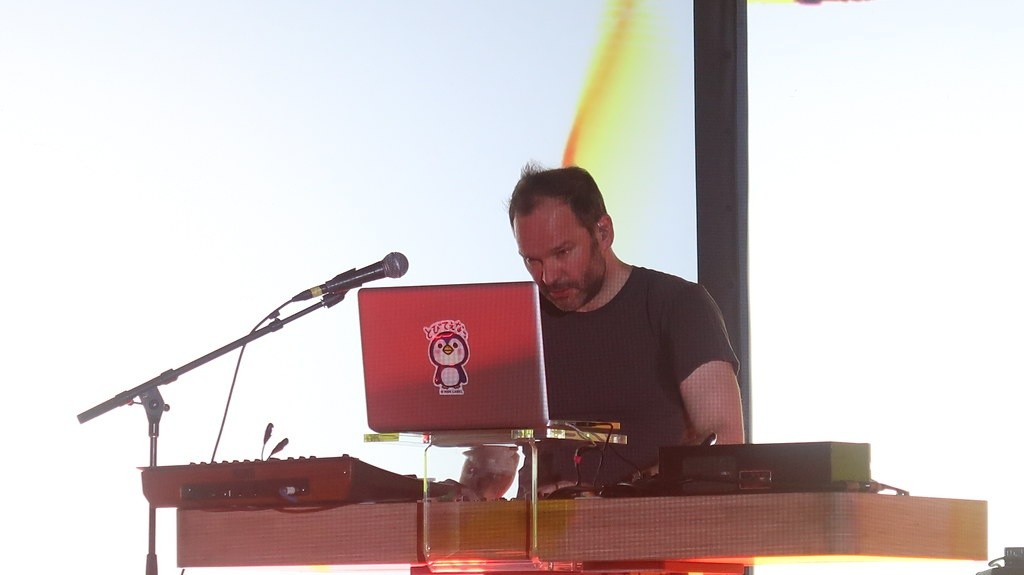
[419,167,744,500]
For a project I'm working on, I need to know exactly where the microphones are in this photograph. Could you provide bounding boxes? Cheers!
[291,252,408,302]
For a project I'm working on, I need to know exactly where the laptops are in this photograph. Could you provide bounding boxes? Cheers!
[359,281,622,432]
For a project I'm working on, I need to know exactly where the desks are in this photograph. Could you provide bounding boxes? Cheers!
[177,493,988,575]
